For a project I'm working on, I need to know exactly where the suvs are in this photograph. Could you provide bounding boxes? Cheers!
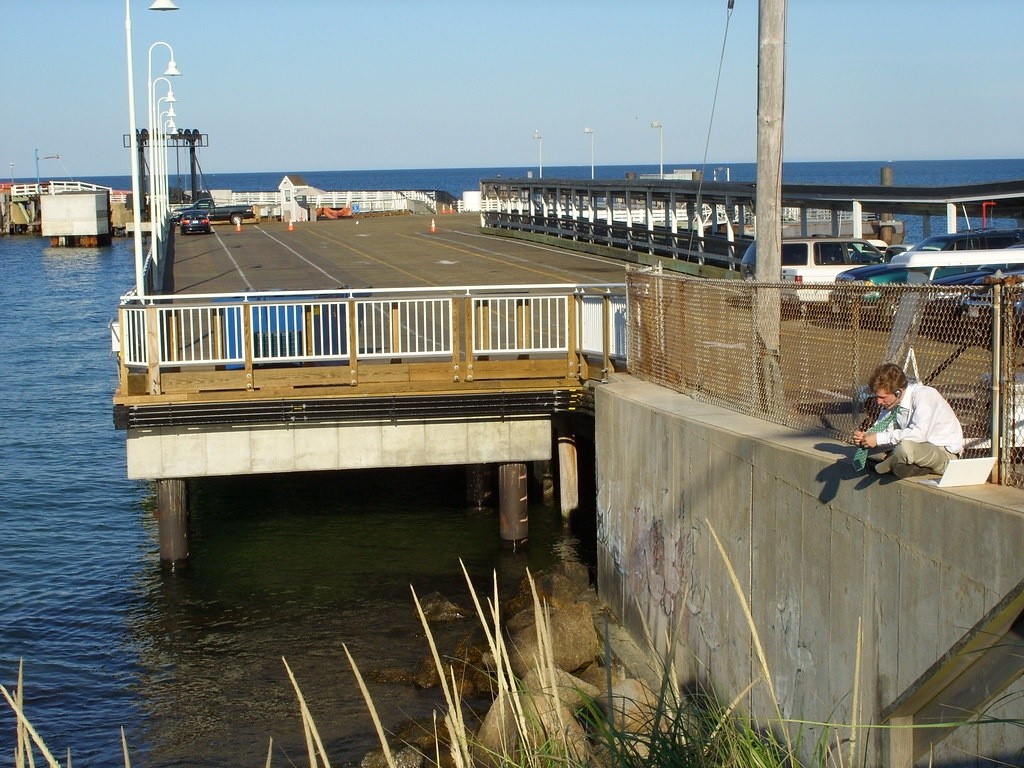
[830,225,1024,328]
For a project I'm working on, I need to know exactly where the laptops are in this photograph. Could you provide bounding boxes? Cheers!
[918,456,997,488]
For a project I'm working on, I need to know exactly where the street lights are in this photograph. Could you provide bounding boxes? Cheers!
[583,127,595,180]
[532,129,543,178]
[650,121,665,178]
[151,74,181,267]
[146,40,184,292]
[126,0,180,305]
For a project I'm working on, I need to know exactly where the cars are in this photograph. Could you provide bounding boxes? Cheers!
[865,235,942,265]
[877,242,1024,349]
[178,210,211,234]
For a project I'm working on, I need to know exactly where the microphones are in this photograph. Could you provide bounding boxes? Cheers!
[883,390,902,410]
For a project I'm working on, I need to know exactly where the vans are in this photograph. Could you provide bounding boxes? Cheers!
[741,235,887,321]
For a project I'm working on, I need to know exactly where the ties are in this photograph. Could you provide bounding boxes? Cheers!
[853,405,900,472]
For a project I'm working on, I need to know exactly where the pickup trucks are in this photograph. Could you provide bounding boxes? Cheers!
[171,198,256,226]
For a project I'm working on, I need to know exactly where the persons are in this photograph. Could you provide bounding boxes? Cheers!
[854,364,963,478]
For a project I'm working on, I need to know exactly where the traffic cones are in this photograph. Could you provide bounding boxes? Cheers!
[431,217,436,233]
[235,220,241,231]
[288,219,294,230]
[442,203,446,214]
[449,202,453,213]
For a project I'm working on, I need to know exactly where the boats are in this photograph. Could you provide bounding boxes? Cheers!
[0,149,152,238]
[600,122,747,229]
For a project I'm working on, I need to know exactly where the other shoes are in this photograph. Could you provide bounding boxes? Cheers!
[892,463,931,479]
